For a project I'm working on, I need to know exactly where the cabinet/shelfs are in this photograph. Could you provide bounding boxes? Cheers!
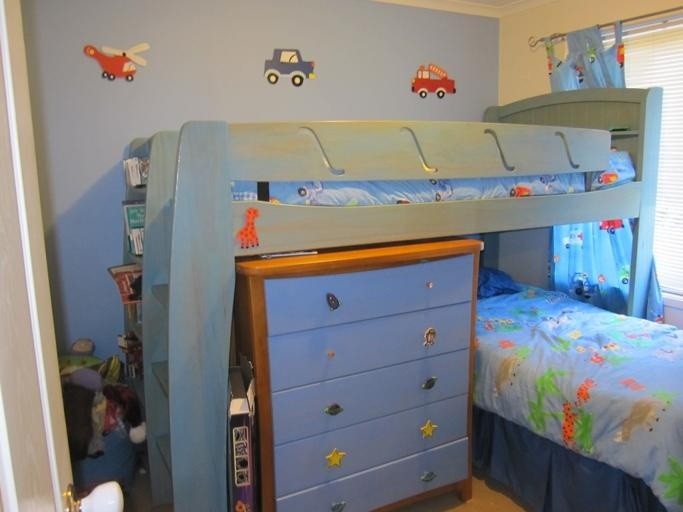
[233,236,485,512]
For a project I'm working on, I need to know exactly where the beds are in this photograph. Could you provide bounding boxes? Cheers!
[136,87,682,511]
